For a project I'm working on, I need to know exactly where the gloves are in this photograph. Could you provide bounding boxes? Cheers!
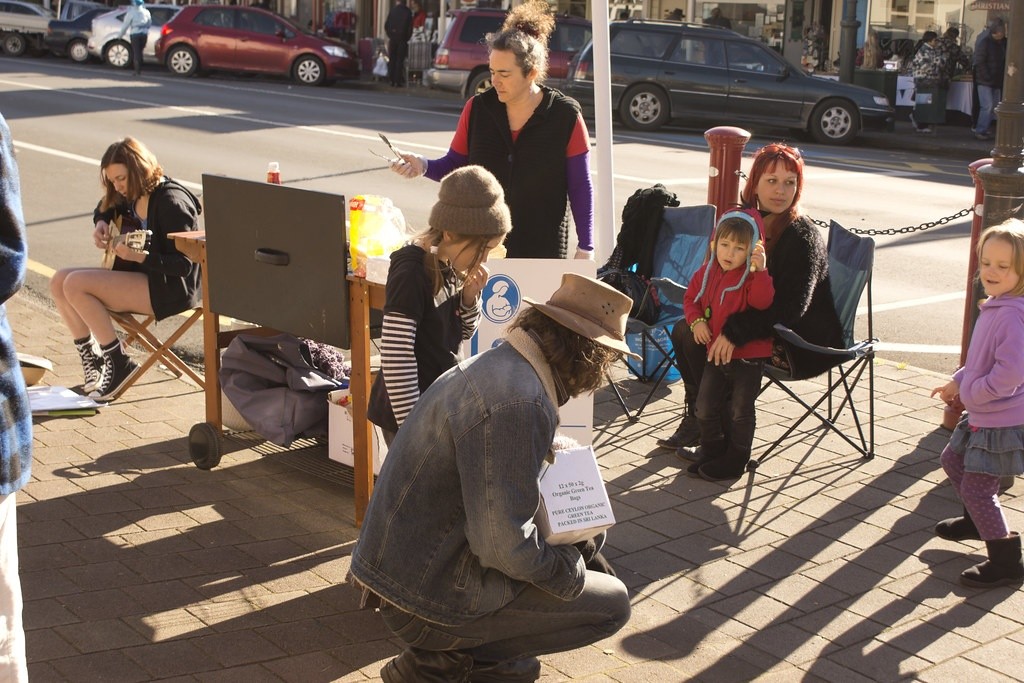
[573,538,595,563]
[583,552,616,575]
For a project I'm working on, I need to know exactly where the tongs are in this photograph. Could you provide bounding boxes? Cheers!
[367,133,406,166]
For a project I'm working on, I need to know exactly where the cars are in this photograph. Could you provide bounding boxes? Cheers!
[0,0,363,87]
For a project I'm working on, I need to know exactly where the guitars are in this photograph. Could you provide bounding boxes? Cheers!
[100,208,153,269]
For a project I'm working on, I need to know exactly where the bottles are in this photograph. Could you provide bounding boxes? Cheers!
[267,162,281,185]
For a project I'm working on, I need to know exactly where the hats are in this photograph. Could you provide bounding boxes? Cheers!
[522,273,643,362]
[692,206,765,304]
[429,165,513,235]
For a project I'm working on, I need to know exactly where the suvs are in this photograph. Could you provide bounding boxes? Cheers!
[558,17,895,146]
[425,6,594,101]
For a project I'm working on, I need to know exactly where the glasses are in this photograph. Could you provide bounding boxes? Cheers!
[761,145,801,162]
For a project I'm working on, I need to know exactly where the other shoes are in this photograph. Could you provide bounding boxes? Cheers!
[686,438,728,477]
[676,444,705,465]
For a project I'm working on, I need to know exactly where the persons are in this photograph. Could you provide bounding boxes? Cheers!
[0,113,32,682]
[349,0,1023,683]
[324,0,358,45]
[49,136,198,402]
[118,0,152,77]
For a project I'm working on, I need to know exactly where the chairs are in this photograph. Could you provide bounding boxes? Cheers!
[746,220,879,471]
[602,204,716,424]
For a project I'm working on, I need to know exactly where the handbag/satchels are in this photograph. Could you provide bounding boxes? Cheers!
[603,269,661,326]
[216,332,347,449]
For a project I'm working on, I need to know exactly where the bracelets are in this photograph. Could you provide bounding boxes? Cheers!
[690,318,707,331]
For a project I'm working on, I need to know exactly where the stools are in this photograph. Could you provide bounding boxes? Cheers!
[106,279,206,401]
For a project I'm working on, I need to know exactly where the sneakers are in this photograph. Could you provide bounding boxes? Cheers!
[88,342,139,400]
[78,336,105,392]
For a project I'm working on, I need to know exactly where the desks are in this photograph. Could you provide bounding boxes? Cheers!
[946,80,1001,127]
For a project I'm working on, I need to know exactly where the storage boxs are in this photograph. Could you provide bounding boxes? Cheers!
[328,389,387,475]
[883,58,902,70]
[538,446,616,547]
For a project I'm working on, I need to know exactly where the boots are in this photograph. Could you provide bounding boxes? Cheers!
[698,419,756,482]
[962,531,1024,588]
[935,502,981,541]
[378,646,541,683]
[656,389,702,450]
